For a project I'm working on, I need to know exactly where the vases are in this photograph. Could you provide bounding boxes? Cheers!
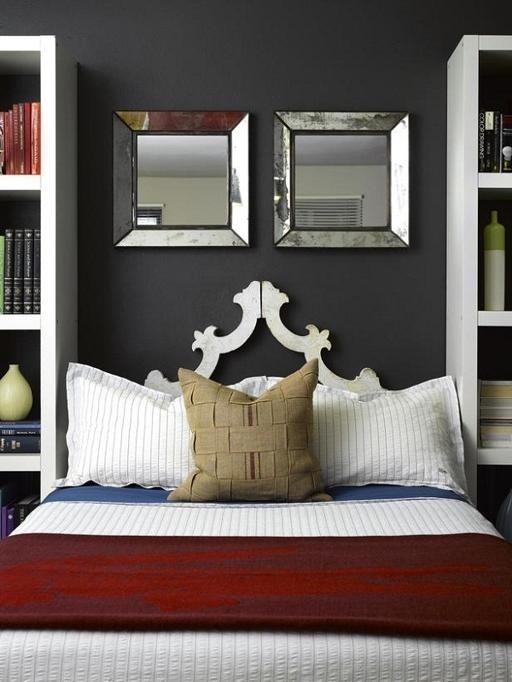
[0,364,33,422]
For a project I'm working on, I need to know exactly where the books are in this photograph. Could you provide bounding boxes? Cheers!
[478,111,511,173]
[0,102,41,540]
[478,381,512,449]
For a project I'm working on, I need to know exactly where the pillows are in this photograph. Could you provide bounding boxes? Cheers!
[48,360,241,493]
[166,357,334,505]
[240,374,477,507]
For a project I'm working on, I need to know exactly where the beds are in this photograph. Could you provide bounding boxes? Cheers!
[0,278,512,681]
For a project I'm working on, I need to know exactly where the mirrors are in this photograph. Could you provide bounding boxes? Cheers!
[111,110,250,248]
[272,110,412,249]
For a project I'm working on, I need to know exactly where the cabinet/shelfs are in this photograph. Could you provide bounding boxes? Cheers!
[445,34,512,508]
[0,34,79,545]
